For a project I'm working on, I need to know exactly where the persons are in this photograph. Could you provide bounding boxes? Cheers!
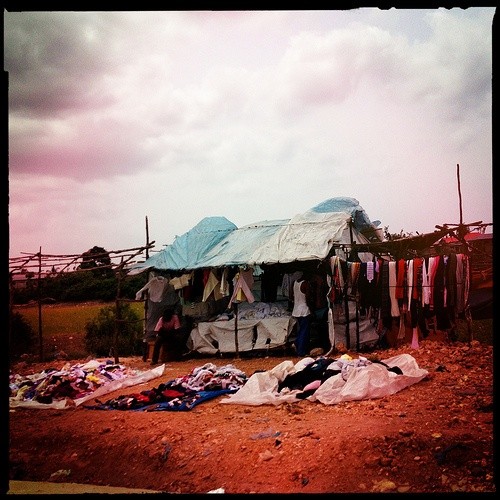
[152,311,185,366]
[290,270,317,357]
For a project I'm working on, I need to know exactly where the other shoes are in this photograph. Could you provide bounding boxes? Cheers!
[154,317,163,331]
[173,315,181,330]
